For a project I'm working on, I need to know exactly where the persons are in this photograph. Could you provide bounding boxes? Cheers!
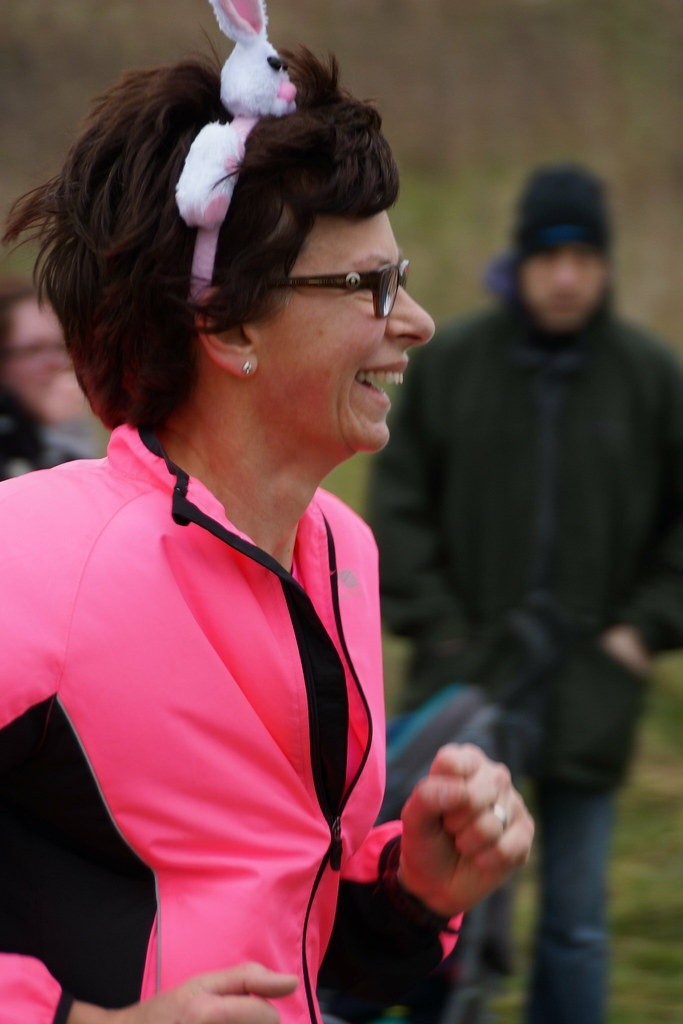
[365,162,683,1023]
[0,55,536,1024]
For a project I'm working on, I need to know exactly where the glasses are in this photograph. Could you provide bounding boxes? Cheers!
[259,259,410,317]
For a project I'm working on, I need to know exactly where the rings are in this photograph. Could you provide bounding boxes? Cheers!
[490,804,508,830]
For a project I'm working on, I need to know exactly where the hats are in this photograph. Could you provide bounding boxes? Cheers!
[517,167,614,254]
